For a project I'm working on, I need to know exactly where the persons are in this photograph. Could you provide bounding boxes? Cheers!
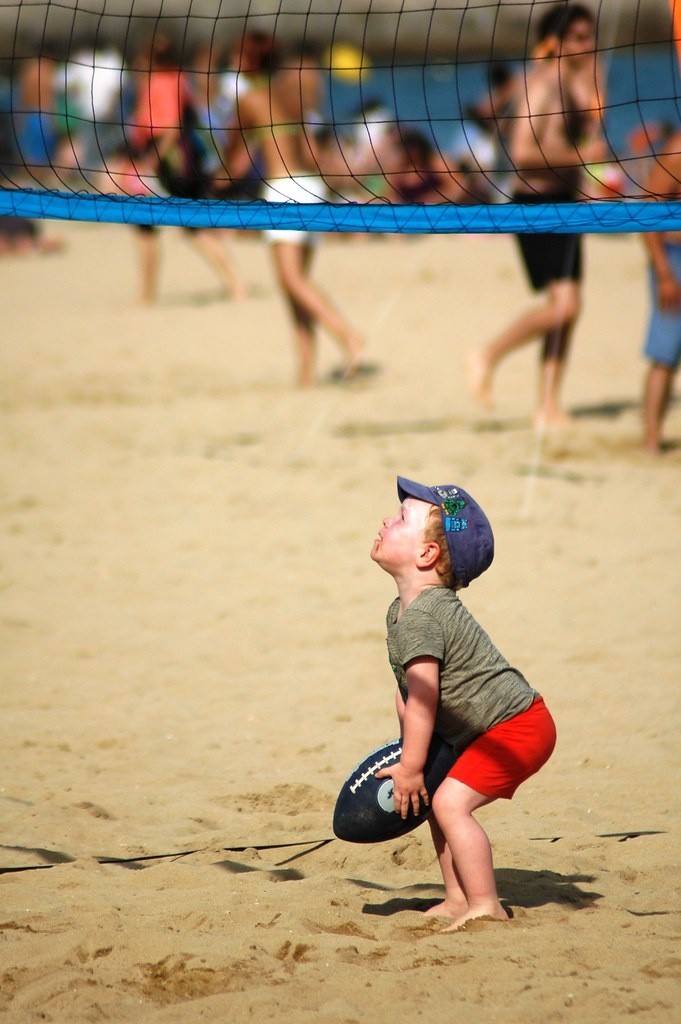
[210,28,369,385]
[471,3,612,434]
[371,474,557,933]
[635,129,681,445]
[1,26,676,302]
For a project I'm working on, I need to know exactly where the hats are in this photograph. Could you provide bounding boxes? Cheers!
[396,476,494,588]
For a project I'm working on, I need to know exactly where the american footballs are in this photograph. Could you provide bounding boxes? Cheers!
[332,737,458,844]
